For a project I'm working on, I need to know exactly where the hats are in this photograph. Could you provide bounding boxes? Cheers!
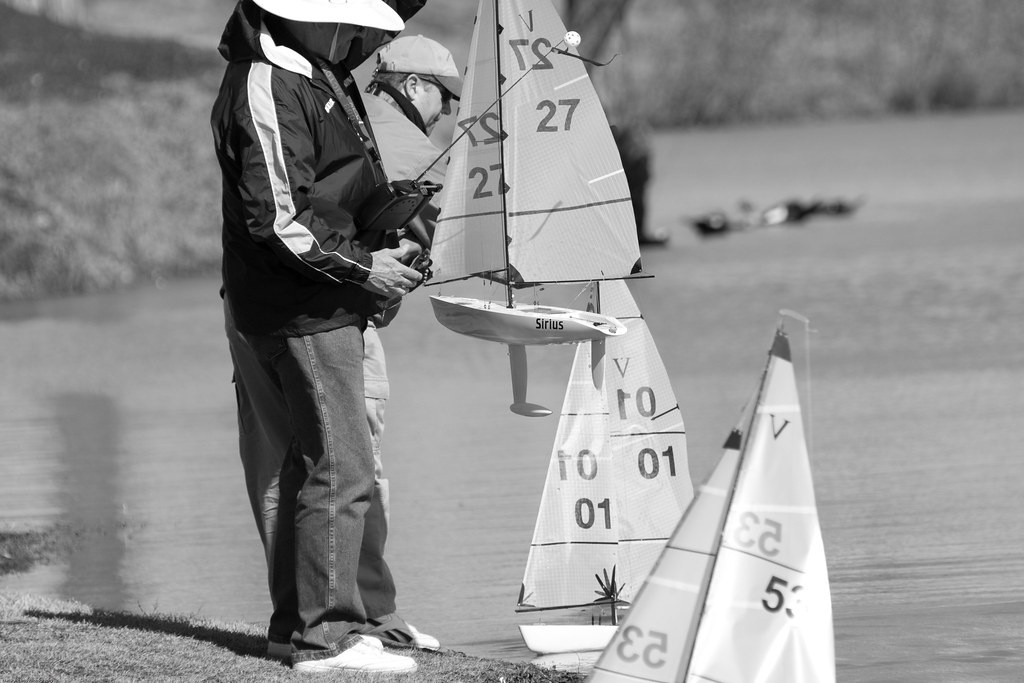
[252,0,407,62]
[376,33,463,100]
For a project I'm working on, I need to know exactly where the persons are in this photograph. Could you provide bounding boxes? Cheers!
[212,0,422,675]
[361,35,462,653]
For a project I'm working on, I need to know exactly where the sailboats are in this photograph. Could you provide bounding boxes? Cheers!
[515,275,695,654]
[431,2,651,352]
[586,312,835,683]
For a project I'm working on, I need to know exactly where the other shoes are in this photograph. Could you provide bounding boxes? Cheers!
[267,635,384,662]
[293,642,416,676]
[357,612,441,652]
